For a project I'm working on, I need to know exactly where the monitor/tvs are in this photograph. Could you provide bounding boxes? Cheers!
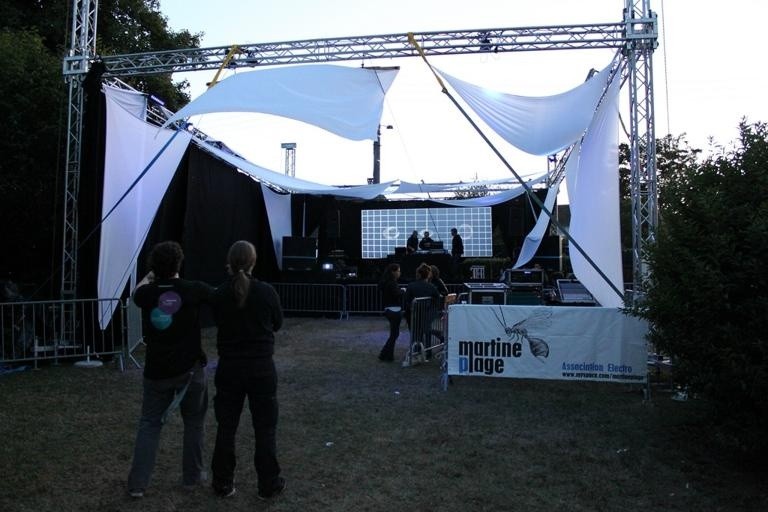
[557,278,596,302]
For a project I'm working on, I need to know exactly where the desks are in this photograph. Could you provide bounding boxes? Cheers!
[462,283,510,304]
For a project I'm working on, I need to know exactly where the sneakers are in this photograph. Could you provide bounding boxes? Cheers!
[126,482,144,498]
[221,478,236,497]
[184,470,207,490]
[257,476,286,500]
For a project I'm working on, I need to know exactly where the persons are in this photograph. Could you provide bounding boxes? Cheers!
[127,239,217,498]
[450,228,464,280]
[424,263,449,361]
[401,261,441,361]
[209,239,286,502]
[419,231,434,250]
[407,230,418,253]
[376,263,405,361]
